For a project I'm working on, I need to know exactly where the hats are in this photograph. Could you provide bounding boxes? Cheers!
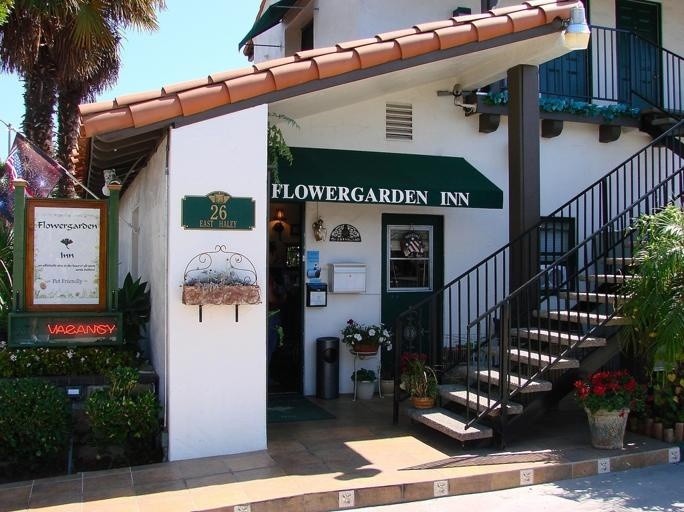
[400,232,424,256]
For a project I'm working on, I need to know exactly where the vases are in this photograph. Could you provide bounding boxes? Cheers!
[352,343,380,357]
[581,406,632,450]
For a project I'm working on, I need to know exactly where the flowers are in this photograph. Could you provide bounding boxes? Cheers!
[337,318,395,351]
[570,365,641,417]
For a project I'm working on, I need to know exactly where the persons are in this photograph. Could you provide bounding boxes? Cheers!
[268,265,290,391]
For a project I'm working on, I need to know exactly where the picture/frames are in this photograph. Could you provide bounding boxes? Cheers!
[25,198,107,312]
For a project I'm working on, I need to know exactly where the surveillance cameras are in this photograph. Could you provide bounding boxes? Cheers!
[452,83,463,97]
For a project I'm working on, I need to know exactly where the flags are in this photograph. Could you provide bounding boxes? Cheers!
[1,131,66,228]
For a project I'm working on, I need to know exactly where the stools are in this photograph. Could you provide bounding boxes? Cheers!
[347,350,384,401]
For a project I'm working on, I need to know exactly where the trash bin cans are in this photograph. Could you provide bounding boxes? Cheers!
[316,337,340,400]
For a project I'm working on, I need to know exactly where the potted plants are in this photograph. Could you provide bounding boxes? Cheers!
[349,367,379,400]
[403,350,440,409]
